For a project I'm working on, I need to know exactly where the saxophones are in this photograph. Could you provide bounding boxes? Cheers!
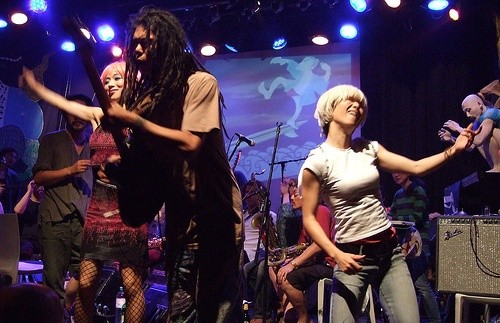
[251,169,272,230]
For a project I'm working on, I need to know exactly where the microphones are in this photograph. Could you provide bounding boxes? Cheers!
[235,132,255,146]
[245,200,271,221]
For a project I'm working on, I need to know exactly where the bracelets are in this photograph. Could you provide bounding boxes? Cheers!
[442,147,453,159]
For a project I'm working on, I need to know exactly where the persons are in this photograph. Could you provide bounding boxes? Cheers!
[0,154,18,212]
[437,95,500,172]
[14,178,40,257]
[3,148,19,169]
[241,179,278,323]
[19,61,145,323]
[30,93,94,299]
[297,85,482,323]
[250,179,302,323]
[104,9,243,323]
[387,172,441,323]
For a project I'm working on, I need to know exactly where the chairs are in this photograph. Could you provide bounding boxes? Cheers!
[0,214,500,323]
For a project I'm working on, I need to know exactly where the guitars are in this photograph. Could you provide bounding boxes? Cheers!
[65,18,166,228]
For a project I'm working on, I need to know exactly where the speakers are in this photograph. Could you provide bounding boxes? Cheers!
[436,215,500,298]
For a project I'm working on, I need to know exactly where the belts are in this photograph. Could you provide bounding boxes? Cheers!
[334,234,399,257]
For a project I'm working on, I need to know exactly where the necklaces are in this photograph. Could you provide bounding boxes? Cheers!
[268,180,334,323]
